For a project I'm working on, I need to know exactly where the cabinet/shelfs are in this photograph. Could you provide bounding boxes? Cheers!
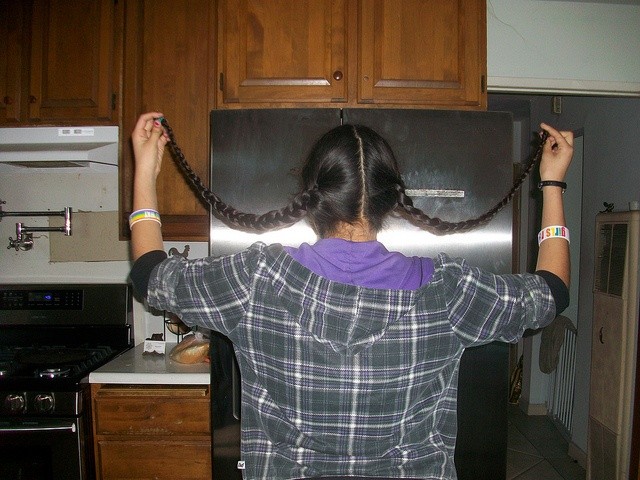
[89,341,211,479]
[120,2,215,242]
[225,1,488,110]
[1,2,116,126]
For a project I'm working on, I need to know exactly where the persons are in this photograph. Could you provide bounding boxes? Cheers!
[130,111,575,479]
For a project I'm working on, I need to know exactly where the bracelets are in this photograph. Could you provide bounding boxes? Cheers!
[128,209,162,230]
[537,225,571,247]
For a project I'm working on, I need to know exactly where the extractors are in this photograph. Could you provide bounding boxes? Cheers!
[0,127,119,170]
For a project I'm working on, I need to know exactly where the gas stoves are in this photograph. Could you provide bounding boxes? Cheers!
[0,341,116,386]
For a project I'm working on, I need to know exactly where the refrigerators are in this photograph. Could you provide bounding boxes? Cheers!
[207,109,514,478]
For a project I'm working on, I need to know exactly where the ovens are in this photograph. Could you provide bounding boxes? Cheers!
[1,418,89,480]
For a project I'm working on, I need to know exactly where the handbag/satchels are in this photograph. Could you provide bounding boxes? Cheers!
[509,355,523,405]
